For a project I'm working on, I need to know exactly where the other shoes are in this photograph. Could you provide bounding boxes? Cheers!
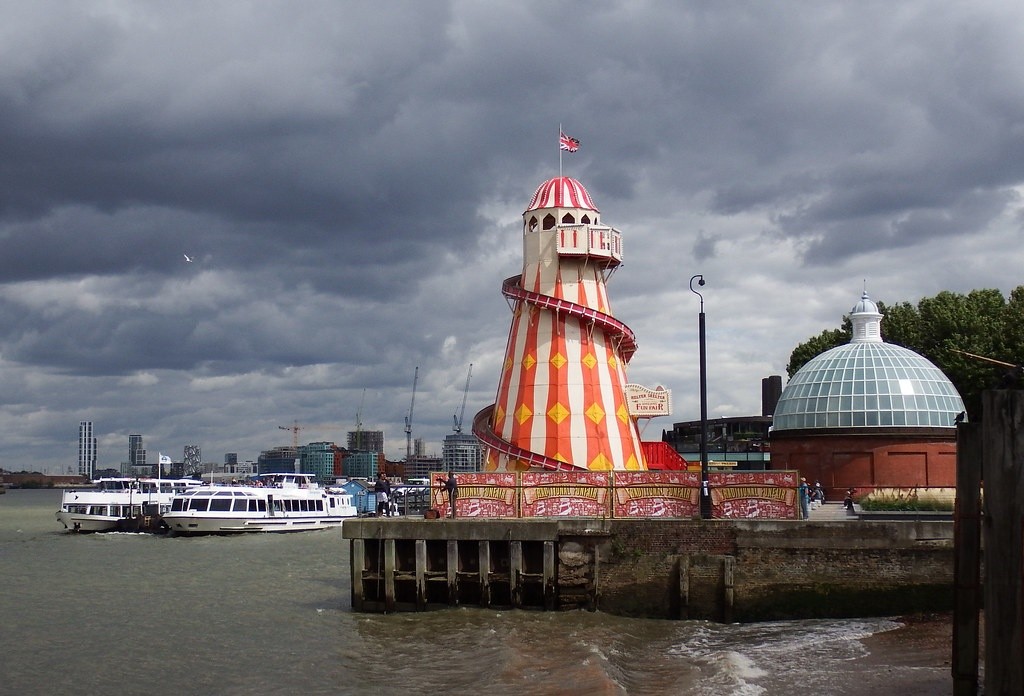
[446,515,456,519]
[377,515,386,518]
[387,514,392,518]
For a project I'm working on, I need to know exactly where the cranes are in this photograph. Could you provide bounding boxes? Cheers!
[452,363,474,434]
[355,387,366,449]
[277,418,305,450]
[404,366,419,458]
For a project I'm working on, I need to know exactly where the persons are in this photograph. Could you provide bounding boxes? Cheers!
[843,487,857,509]
[199,477,281,489]
[799,475,824,520]
[373,472,392,519]
[436,470,459,519]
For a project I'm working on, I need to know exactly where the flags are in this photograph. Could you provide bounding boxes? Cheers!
[559,131,580,153]
[160,454,172,465]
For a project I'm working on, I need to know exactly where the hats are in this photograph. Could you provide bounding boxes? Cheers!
[801,477,806,481]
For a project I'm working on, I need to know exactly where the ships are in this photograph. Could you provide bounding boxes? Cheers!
[58,452,430,537]
